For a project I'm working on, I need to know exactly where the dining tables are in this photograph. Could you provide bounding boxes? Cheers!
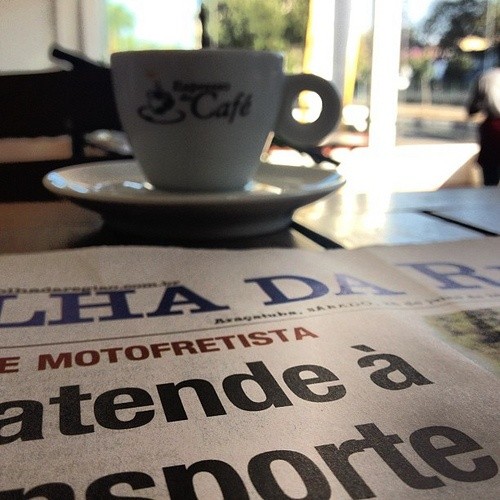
[0,185,500,499]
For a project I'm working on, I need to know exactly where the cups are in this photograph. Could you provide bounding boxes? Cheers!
[111,49,341,197]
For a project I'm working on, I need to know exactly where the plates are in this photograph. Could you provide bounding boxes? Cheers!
[42,159,346,239]
